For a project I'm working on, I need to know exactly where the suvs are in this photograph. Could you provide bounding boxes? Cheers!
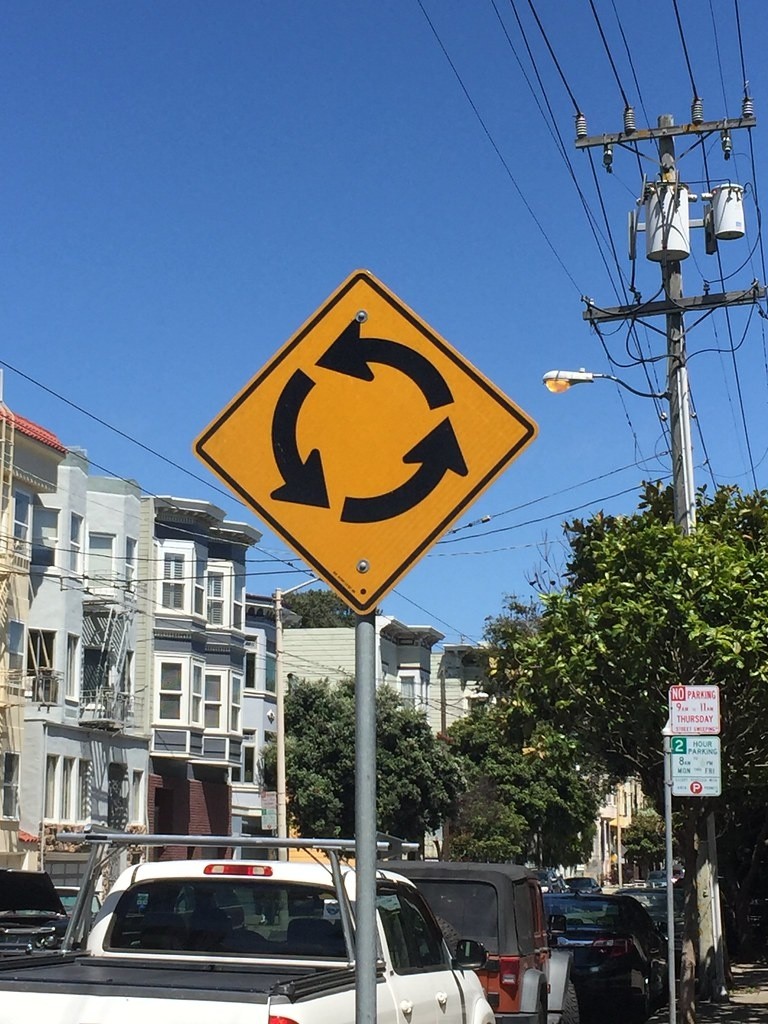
[369,861,582,1024]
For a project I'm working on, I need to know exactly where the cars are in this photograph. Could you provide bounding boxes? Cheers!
[539,892,677,1022]
[563,877,602,895]
[0,869,107,957]
[532,868,571,894]
[672,865,685,880]
[643,871,668,888]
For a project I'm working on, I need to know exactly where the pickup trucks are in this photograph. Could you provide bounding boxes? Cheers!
[1,834,493,1024]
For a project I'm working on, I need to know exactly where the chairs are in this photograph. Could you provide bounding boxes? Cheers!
[129,911,346,957]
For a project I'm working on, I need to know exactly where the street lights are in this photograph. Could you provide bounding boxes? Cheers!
[542,369,728,1003]
